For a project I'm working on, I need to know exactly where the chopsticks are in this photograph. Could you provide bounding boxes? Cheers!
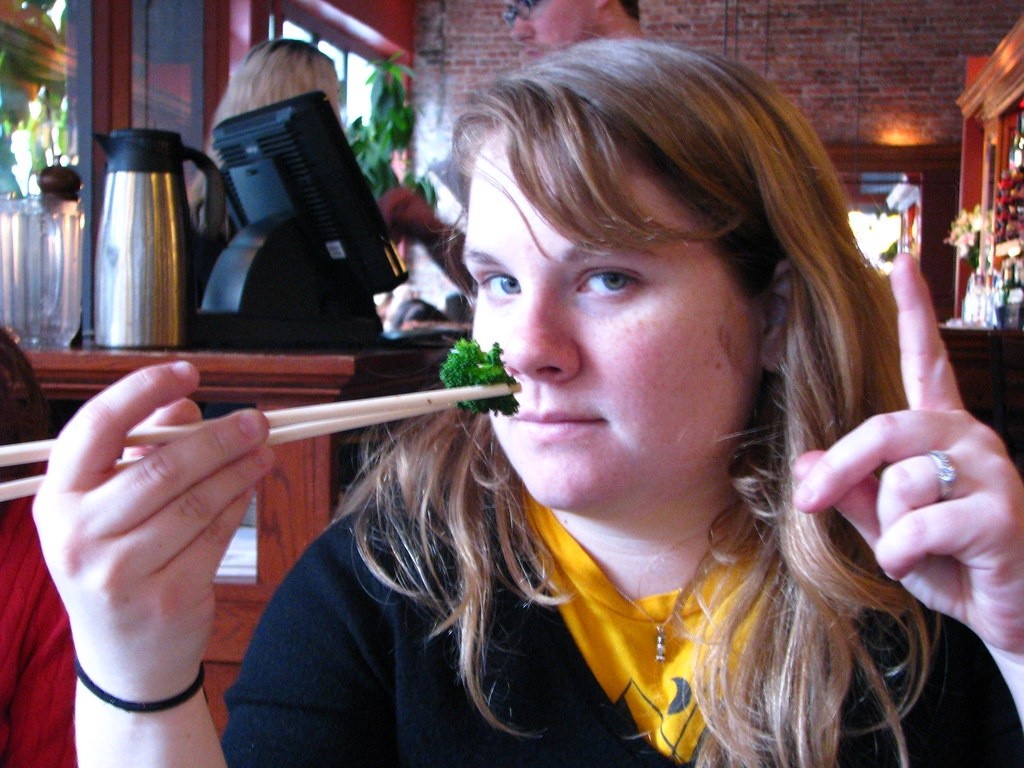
[0,382,521,502]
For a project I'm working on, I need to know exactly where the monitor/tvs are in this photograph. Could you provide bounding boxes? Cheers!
[194,87,409,350]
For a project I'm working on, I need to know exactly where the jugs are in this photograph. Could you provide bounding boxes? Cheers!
[91,127,226,349]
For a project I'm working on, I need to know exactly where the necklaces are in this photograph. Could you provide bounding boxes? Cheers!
[612,549,720,664]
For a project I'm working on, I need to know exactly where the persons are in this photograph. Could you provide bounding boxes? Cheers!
[30,1,1024,768]
[0,326,78,768]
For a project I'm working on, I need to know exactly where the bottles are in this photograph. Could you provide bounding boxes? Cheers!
[962,259,1024,329]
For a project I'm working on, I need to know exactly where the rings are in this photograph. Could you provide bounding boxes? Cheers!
[927,450,956,503]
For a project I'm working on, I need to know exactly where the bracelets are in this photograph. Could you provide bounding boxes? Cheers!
[73,651,208,714]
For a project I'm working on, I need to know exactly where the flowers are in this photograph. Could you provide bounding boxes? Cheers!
[943,204,994,269]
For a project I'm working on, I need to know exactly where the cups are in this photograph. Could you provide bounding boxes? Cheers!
[0,196,85,349]
